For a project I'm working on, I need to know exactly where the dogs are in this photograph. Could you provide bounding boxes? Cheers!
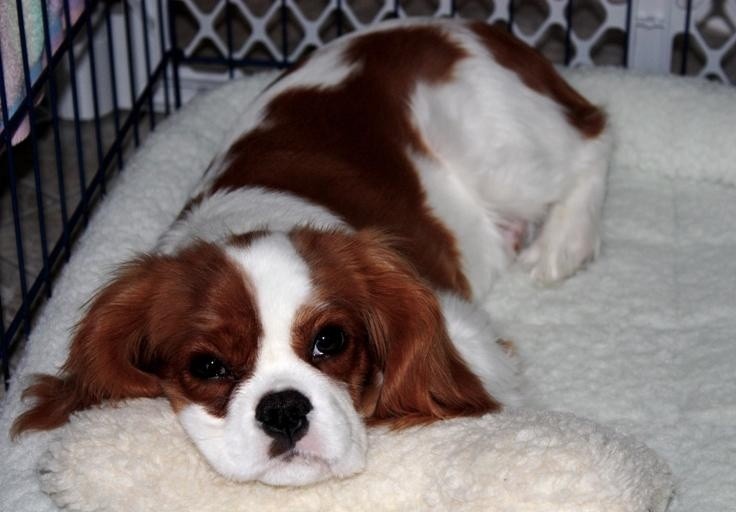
[9,16,617,490]
